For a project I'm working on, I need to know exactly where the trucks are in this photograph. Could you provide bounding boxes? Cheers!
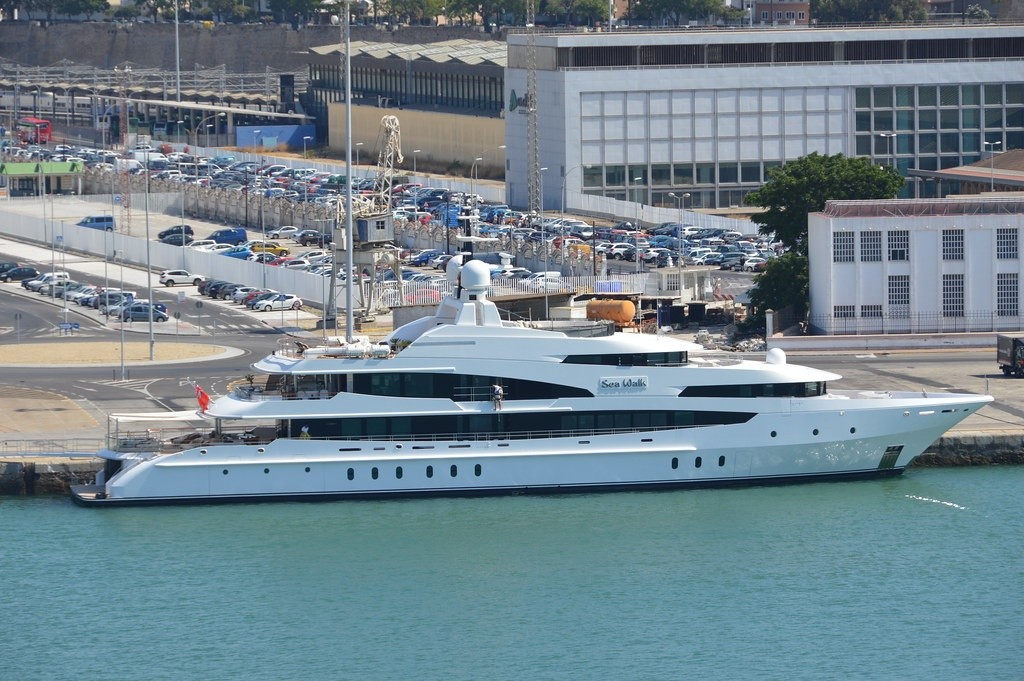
[998,335,1024,379]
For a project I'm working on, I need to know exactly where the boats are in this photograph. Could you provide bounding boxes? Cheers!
[67,251,998,504]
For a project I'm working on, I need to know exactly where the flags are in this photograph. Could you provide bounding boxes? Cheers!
[195,386,212,414]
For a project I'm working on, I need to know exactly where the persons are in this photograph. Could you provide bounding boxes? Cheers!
[492,383,506,410]
[299,425,310,439]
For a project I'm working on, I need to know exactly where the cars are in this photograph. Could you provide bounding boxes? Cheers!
[0,115,796,324]
